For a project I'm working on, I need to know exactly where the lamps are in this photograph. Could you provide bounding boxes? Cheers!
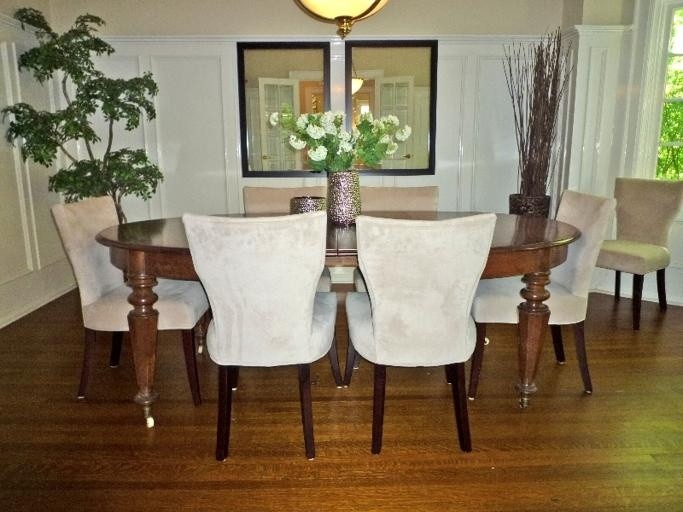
[291,0,390,37]
[349,55,365,94]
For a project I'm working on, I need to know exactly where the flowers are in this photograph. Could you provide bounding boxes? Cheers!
[266,105,414,176]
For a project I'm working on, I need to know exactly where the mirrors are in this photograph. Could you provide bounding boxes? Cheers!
[235,40,331,178]
[345,40,440,176]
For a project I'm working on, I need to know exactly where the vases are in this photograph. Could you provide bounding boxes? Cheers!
[509,192,550,220]
[327,169,361,226]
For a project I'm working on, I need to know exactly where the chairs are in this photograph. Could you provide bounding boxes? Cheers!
[466,189,616,401]
[177,209,344,462]
[242,185,334,361]
[595,177,682,316]
[344,213,497,453]
[49,193,210,407]
[351,186,443,373]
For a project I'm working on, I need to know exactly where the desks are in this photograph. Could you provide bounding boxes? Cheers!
[92,212,581,407]
